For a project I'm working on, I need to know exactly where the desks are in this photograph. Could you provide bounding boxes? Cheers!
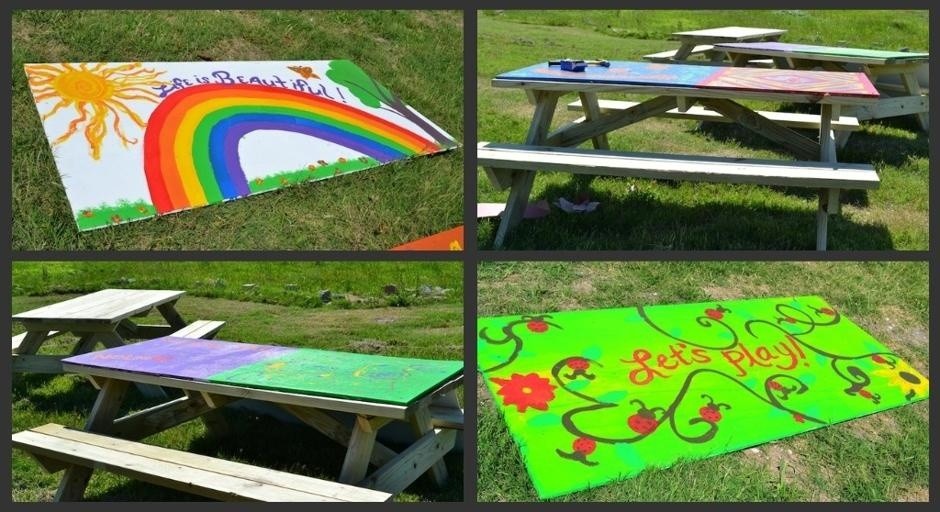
[10,288,190,401]
[491,25,930,254]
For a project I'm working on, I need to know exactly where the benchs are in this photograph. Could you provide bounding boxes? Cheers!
[11,319,465,501]
[473,140,881,250]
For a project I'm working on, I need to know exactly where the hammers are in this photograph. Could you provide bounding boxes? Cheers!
[548,58,610,67]
